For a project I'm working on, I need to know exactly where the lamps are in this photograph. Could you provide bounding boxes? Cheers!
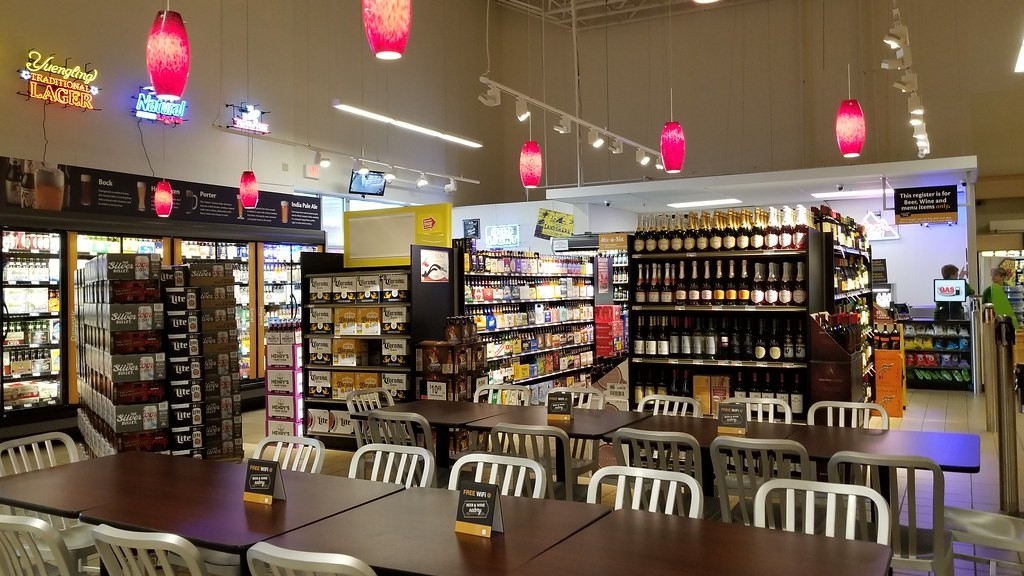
[315,148,456,194]
[145,0,191,102]
[660,0,685,174]
[835,0,864,158]
[155,122,172,218]
[238,0,258,209]
[361,0,412,60]
[476,76,668,171]
[518,1,543,188]
[880,22,931,158]
[329,98,486,150]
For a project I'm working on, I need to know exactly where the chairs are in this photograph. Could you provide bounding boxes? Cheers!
[0,380,1023,576]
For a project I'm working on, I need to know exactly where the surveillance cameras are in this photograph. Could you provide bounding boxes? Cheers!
[605,201,610,207]
[837,184,843,190]
[961,179,966,186]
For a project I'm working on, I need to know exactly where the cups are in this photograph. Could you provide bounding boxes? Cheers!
[80,175,92,206]
[35,169,64,212]
[137,181,146,212]
[281,201,289,223]
[185,190,198,214]
[237,195,245,219]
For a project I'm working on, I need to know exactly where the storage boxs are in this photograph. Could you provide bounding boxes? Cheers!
[70,253,245,460]
[306,277,412,439]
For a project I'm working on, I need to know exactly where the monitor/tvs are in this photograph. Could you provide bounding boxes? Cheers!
[349,169,386,196]
[934,279,966,302]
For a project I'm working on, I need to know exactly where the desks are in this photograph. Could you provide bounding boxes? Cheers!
[350,398,520,488]
[79,464,406,576]
[602,416,798,522]
[261,486,613,576]
[465,406,652,502]
[772,425,981,541]
[506,508,892,576]
[0,449,219,521]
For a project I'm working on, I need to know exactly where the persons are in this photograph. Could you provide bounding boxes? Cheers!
[936,265,975,320]
[982,267,1009,304]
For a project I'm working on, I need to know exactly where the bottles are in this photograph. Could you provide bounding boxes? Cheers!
[181,240,248,261]
[49,290,60,312]
[633,368,805,418]
[2,258,50,283]
[6,157,35,210]
[2,232,60,254]
[439,316,478,454]
[591,351,629,384]
[816,296,901,403]
[264,244,318,262]
[232,262,301,378]
[77,234,164,256]
[59,165,71,208]
[626,442,816,482]
[464,250,592,406]
[597,249,629,315]
[632,205,869,253]
[632,306,809,365]
[2,320,61,406]
[634,255,872,305]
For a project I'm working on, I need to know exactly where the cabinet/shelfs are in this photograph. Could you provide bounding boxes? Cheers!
[299,244,453,464]
[459,203,971,450]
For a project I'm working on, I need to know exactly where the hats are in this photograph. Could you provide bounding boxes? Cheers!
[991,268,1006,276]
